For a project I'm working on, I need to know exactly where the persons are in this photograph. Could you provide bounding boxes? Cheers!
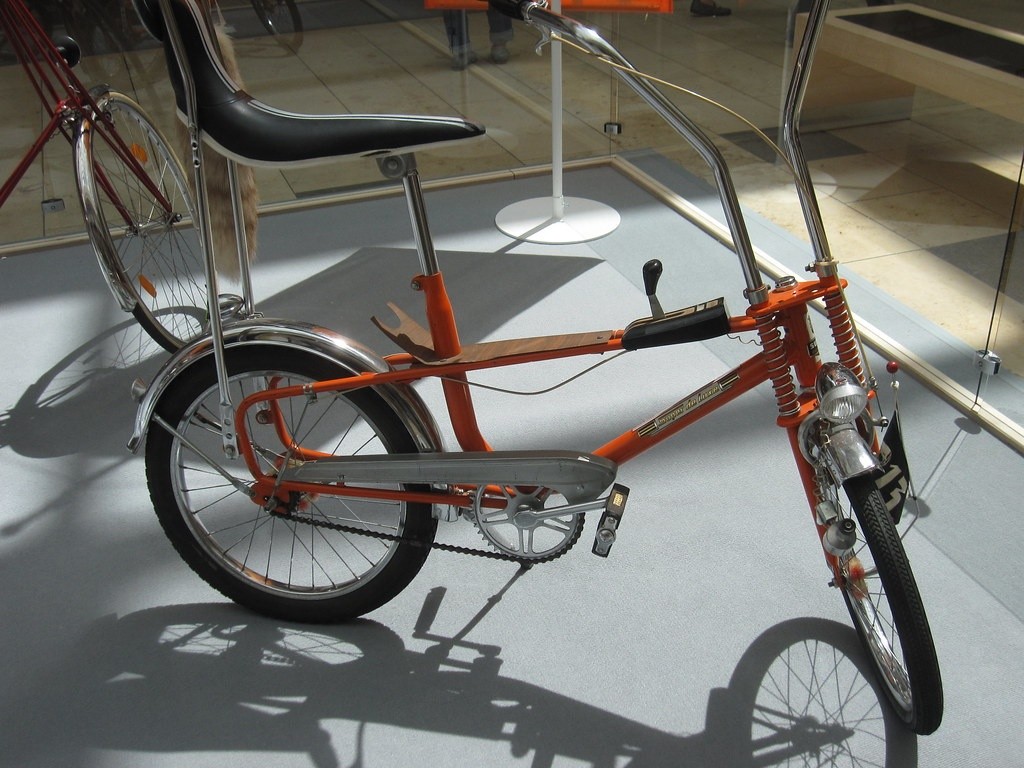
[443,8,514,70]
[691,0,732,16]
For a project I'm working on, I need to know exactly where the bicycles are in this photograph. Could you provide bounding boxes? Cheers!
[0,0,218,355]
[126,0,945,735]
[89,0,304,84]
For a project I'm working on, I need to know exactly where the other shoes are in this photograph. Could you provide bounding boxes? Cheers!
[451,51,477,70]
[491,45,509,64]
